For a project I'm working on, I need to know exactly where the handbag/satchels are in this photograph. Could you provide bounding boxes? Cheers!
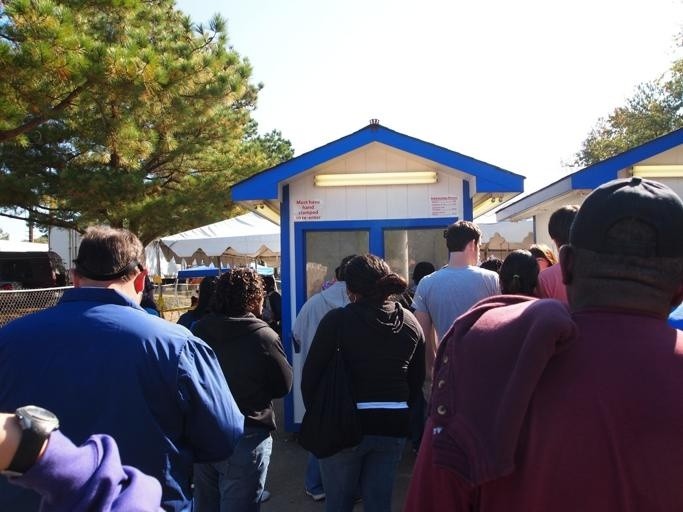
[301,309,364,459]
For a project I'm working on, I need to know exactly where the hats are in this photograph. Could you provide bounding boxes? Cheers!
[569,176,683,256]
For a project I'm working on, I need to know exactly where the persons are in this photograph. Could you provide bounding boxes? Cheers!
[535,205,577,312]
[1,406,167,512]
[527,242,555,272]
[500,249,540,296]
[412,221,500,378]
[189,270,294,512]
[260,275,283,325]
[1,228,244,512]
[301,254,427,512]
[177,276,218,330]
[286,256,358,368]
[411,260,435,290]
[404,176,682,511]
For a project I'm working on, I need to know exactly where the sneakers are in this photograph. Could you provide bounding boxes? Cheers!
[261,491,270,502]
[305,486,325,500]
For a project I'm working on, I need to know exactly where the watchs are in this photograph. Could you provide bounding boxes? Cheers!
[2,405,60,477]
